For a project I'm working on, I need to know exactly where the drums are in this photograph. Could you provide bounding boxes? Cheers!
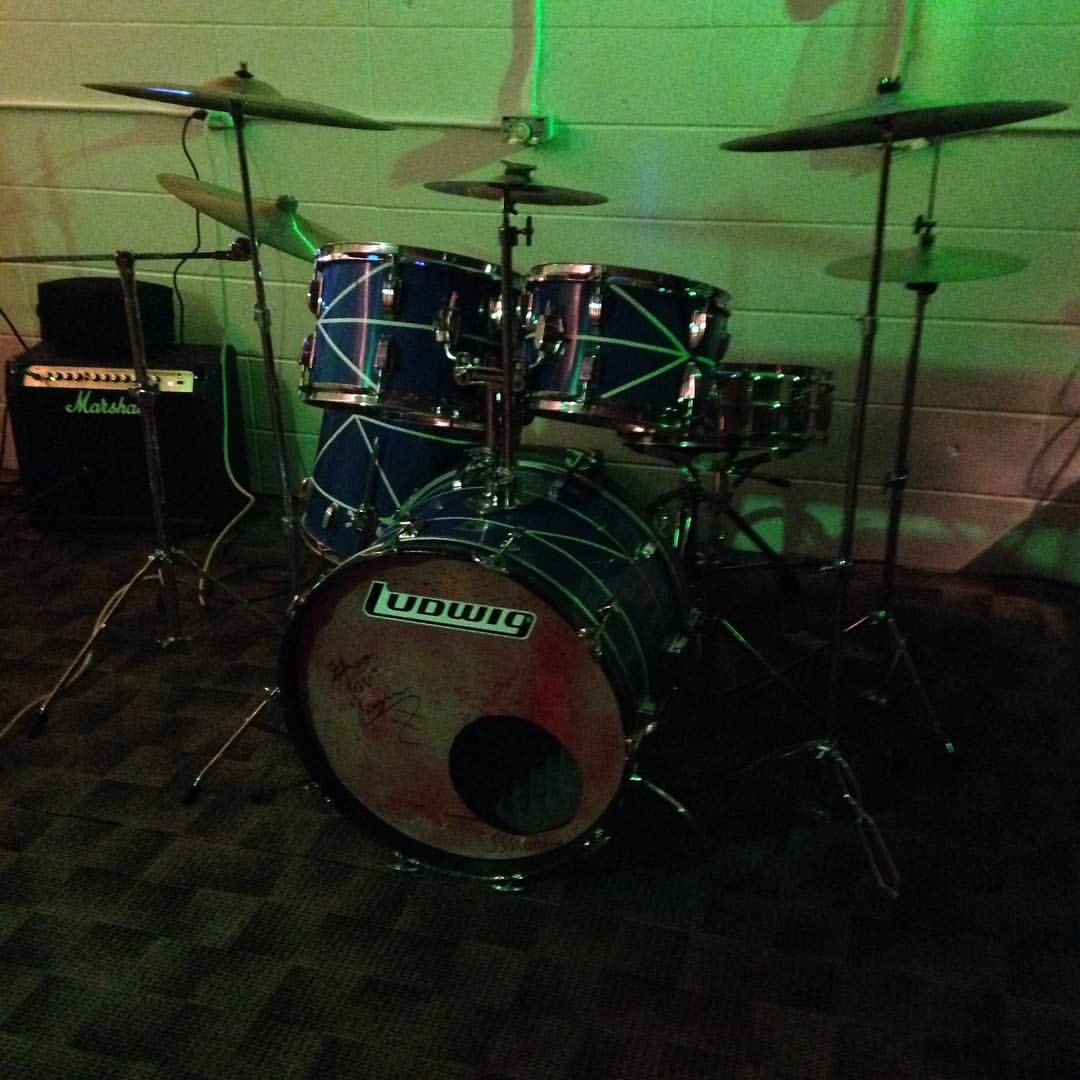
[297,405,521,571]
[275,442,709,884]
[523,258,734,427]
[620,359,841,463]
[300,238,528,434]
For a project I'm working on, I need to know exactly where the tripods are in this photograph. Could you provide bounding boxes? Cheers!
[0,62,961,901]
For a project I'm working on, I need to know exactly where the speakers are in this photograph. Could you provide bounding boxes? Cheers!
[37,276,175,354]
[6,342,249,520]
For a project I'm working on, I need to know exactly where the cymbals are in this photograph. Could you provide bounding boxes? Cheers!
[822,245,1028,287]
[79,74,400,135]
[420,177,611,208]
[717,86,1072,156]
[154,169,354,266]
